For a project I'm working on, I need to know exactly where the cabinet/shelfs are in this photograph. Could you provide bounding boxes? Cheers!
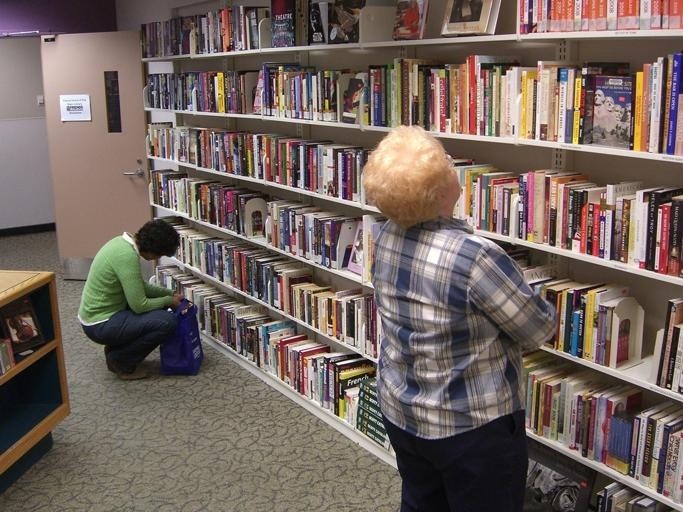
[138,5,682,511]
[0,262,71,495]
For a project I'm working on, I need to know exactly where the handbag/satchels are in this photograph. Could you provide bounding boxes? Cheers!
[157,306,205,376]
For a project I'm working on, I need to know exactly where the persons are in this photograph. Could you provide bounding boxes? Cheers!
[79,220,187,383]
[362,126,558,512]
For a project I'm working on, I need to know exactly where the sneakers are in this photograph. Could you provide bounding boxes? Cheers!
[104,346,118,373]
[118,368,147,380]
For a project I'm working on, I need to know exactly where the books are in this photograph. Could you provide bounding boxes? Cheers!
[140,0,683,156]
[451,159,683,512]
[147,122,395,454]
[0,302,47,375]
